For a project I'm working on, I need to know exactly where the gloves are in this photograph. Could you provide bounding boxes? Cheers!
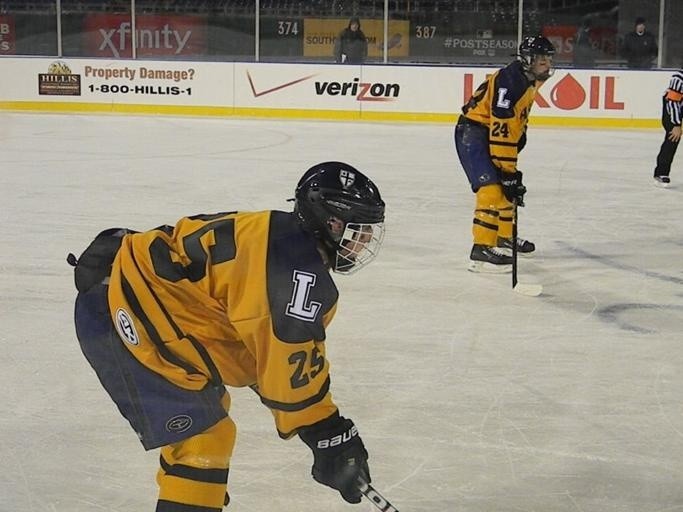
[500,168,527,208]
[296,417,373,505]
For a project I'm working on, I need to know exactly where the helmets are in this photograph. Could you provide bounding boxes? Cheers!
[293,159,387,226]
[518,34,557,56]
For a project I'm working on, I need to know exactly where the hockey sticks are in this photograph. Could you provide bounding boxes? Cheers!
[510,198,543,296]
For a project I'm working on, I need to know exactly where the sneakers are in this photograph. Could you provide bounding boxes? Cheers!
[653,174,670,184]
[469,242,514,265]
[495,234,536,254]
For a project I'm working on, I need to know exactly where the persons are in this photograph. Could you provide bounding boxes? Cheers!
[67,161,389,508]
[332,17,368,62]
[652,67,682,188]
[452,33,557,272]
[620,16,658,68]
[573,19,595,67]
